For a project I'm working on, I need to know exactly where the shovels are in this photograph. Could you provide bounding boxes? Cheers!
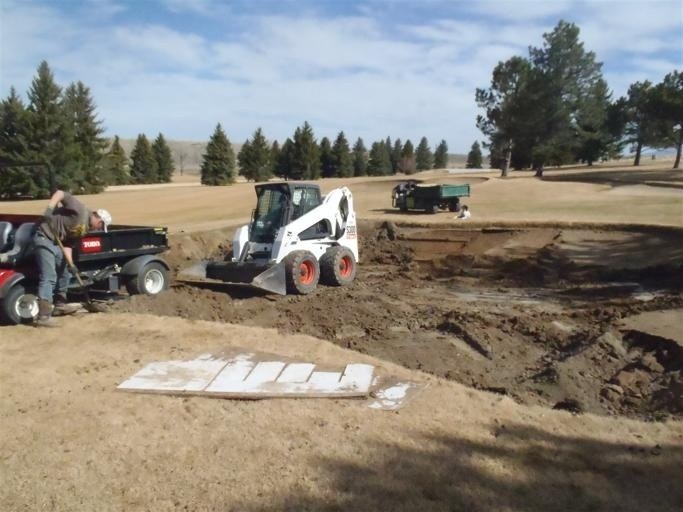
[47,221,110,312]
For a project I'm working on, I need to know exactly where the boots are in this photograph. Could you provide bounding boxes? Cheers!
[35,300,64,329]
[51,296,81,317]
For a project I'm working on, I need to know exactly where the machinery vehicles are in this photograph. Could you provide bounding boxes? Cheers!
[172,181,359,298]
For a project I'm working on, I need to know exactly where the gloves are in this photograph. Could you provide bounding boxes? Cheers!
[43,204,55,225]
[66,263,79,276]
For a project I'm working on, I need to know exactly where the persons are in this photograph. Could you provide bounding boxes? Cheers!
[263,194,299,230]
[30,190,112,328]
[455,204,471,220]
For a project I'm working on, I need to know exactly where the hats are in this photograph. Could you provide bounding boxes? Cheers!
[95,207,113,234]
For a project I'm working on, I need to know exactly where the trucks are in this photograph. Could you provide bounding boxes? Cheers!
[392,183,470,213]
[0,214,170,324]
